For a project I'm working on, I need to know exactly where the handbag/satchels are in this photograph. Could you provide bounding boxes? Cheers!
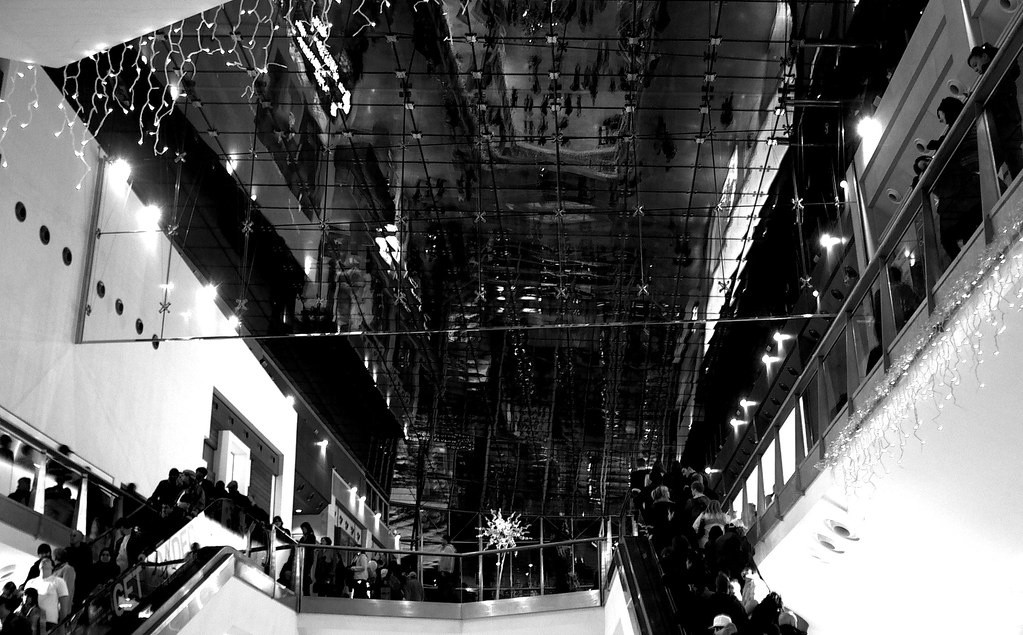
[697,511,706,538]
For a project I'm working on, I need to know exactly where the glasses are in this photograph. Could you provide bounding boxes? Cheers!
[102,553,109,557]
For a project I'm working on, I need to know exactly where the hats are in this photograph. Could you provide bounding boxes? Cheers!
[228,481,237,488]
[58,445,74,454]
[708,614,732,629]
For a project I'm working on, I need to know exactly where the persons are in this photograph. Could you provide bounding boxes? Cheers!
[927,97,1008,223]
[296,522,400,600]
[967,42,1023,182]
[0,434,291,635]
[912,156,1008,262]
[404,571,424,601]
[438,535,457,602]
[875,266,923,354]
[629,458,809,635]
[509,38,734,233]
